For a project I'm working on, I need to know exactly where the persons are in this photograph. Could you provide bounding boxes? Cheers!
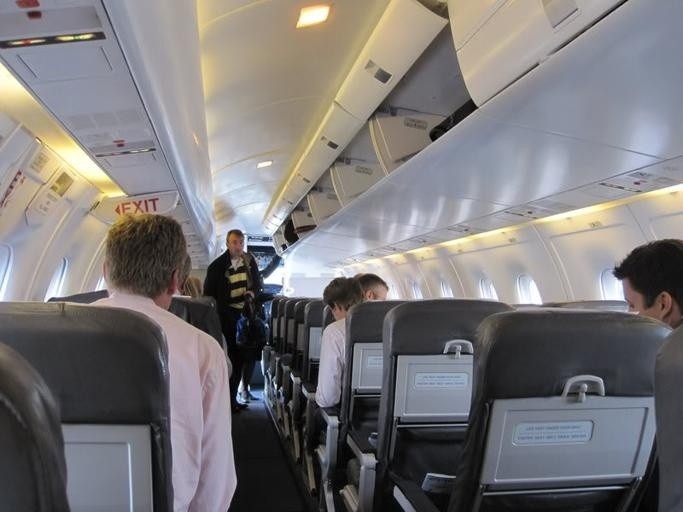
[173,254,233,379]
[315,276,370,408]
[183,274,201,297]
[241,251,286,403]
[203,229,261,413]
[612,238,683,329]
[353,273,364,281]
[87,212,238,512]
[359,273,388,301]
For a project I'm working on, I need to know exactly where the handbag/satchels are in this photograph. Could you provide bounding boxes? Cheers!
[236,295,268,350]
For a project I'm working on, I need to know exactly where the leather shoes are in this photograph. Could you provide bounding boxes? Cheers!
[231,391,260,413]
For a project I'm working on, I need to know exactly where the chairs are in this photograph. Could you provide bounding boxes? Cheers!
[260,291,682,508]
[1,291,244,508]
